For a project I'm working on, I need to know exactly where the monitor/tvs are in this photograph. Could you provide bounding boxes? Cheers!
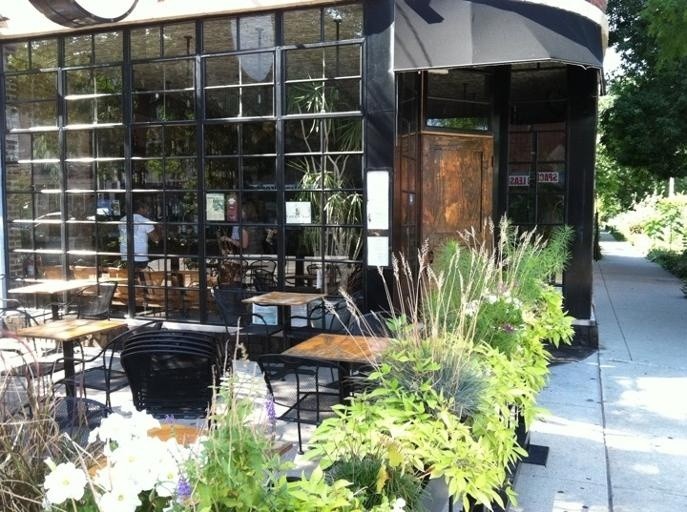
[97,198,122,218]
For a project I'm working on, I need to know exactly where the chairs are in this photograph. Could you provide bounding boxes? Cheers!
[0,228,421,495]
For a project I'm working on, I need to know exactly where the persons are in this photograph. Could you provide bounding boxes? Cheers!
[113,194,304,269]
